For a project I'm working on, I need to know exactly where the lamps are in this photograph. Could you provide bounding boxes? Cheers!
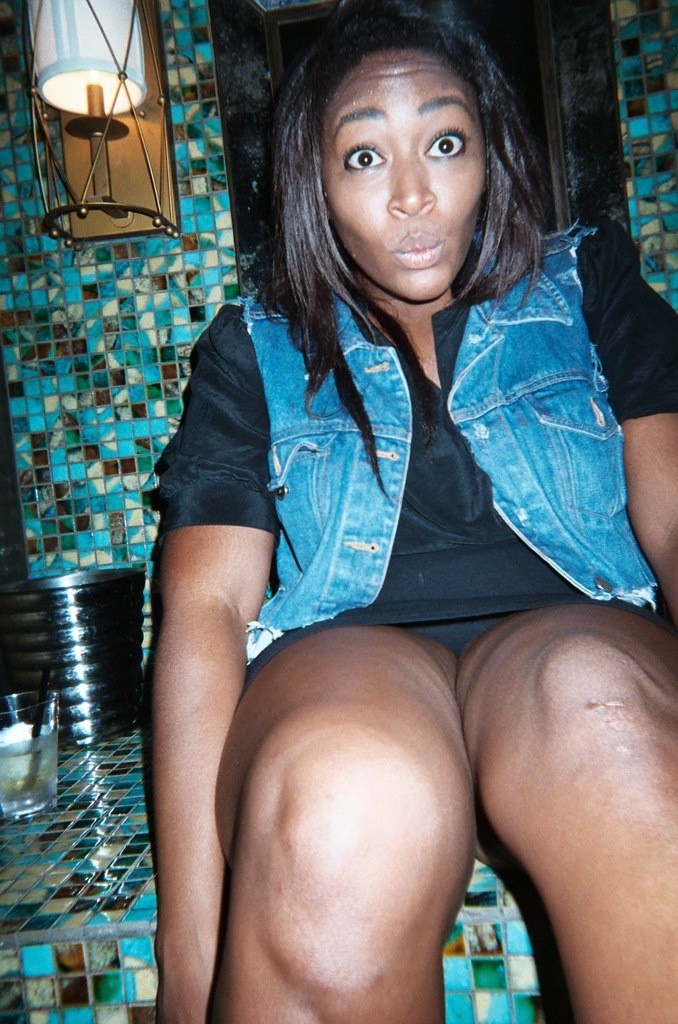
[20,0,183,252]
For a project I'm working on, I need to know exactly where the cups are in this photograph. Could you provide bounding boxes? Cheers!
[0,691,60,822]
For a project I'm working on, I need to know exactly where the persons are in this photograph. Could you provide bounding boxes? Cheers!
[146,9,678,1024]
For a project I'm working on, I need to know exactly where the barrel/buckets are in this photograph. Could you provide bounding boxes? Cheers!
[0,567,147,749]
